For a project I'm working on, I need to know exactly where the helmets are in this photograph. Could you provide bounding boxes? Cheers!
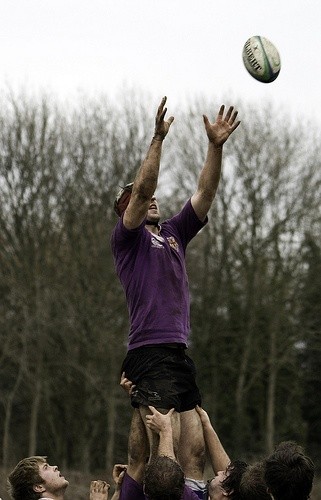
[112,178,143,215]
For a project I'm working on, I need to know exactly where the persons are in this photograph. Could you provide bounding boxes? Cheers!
[241,440,274,500]
[146,403,249,500]
[119,372,202,500]
[111,95,242,500]
[7,455,129,500]
[262,441,315,499]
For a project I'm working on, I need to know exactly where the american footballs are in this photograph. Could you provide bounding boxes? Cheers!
[243,35,281,84]
